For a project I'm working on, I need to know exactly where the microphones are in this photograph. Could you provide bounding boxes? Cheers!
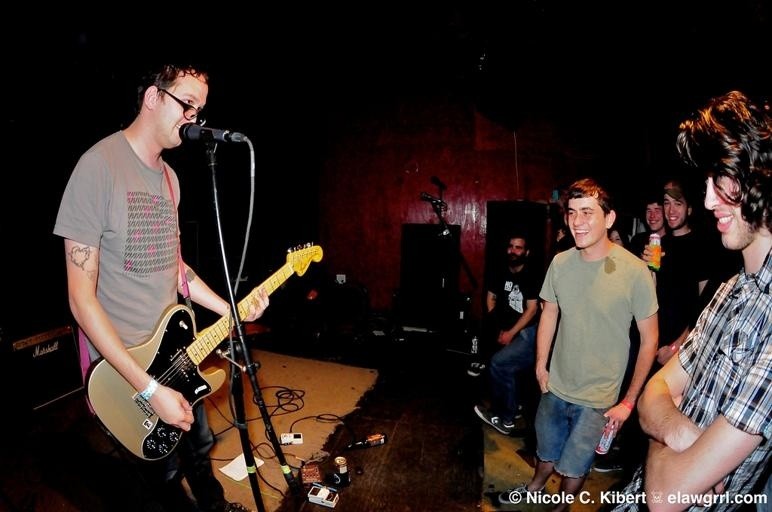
[177,121,234,144]
[418,190,448,209]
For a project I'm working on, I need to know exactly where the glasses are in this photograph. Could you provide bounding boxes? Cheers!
[160,88,203,120]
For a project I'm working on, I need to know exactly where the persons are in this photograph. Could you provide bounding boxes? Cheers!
[53,58,252,512]
[608,229,629,251]
[630,196,667,257]
[554,237,573,254]
[475,236,539,436]
[498,178,657,512]
[597,90,771,511]
[591,181,708,473]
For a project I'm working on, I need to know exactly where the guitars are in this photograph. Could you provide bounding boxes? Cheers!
[85,242,324,463]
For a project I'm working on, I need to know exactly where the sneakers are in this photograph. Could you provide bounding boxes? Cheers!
[224,502,254,512]
[467,362,486,376]
[474,406,514,434]
[499,485,545,504]
[514,405,522,418]
[595,448,623,473]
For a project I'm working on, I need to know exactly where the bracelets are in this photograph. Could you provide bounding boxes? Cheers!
[139,379,159,401]
[620,399,634,410]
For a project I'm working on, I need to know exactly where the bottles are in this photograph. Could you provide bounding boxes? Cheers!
[595,420,615,454]
[347,431,388,449]
[648,233,661,270]
[332,456,351,487]
